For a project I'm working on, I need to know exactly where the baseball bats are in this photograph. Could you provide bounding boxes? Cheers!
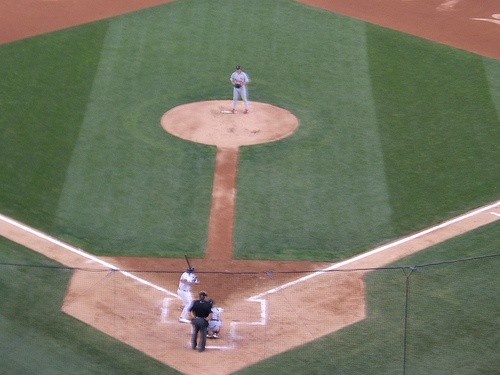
[184,254,191,269]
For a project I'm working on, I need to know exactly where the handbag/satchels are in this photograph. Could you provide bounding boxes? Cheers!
[204,317,209,327]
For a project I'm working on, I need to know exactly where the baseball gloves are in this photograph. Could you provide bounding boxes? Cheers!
[235,84,241,88]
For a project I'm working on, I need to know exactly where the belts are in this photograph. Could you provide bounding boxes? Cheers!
[178,288,187,291]
[210,318,219,321]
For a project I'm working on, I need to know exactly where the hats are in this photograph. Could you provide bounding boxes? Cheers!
[236,65,240,69]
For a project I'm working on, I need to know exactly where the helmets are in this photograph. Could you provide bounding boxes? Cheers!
[187,266,194,273]
[200,291,207,298]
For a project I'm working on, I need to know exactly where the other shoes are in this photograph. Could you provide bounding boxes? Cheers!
[213,335,218,338]
[232,110,235,113]
[245,110,248,113]
[180,318,188,322]
[206,335,211,338]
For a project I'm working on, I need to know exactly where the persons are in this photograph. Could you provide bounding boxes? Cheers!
[177,267,200,323]
[230,65,250,113]
[187,291,224,353]
